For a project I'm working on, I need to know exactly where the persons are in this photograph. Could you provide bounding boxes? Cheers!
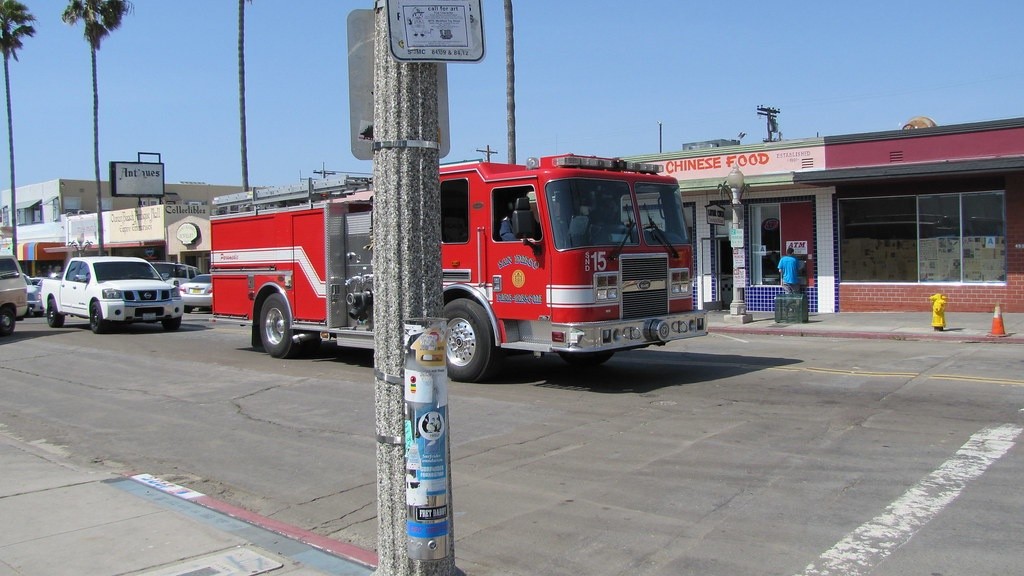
[777,248,806,318]
[499,212,537,243]
[589,200,629,246]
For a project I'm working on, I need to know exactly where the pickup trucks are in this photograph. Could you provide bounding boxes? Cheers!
[40,256,184,333]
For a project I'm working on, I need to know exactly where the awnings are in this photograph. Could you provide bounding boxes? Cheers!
[39,196,58,205]
[15,199,42,212]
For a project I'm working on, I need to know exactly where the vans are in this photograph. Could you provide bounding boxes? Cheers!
[0,254,28,338]
[149,262,202,289]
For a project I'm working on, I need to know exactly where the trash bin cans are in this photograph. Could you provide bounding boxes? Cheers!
[775,293,809,324]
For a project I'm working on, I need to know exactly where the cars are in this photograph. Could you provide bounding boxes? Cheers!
[23,272,65,318]
[179,273,213,315]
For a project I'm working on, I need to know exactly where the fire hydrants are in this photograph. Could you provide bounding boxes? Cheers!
[929,293,949,331]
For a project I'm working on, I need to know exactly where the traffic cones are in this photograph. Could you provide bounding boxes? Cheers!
[987,303,1008,337]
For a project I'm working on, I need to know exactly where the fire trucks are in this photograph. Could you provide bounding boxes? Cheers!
[209,154,708,385]
